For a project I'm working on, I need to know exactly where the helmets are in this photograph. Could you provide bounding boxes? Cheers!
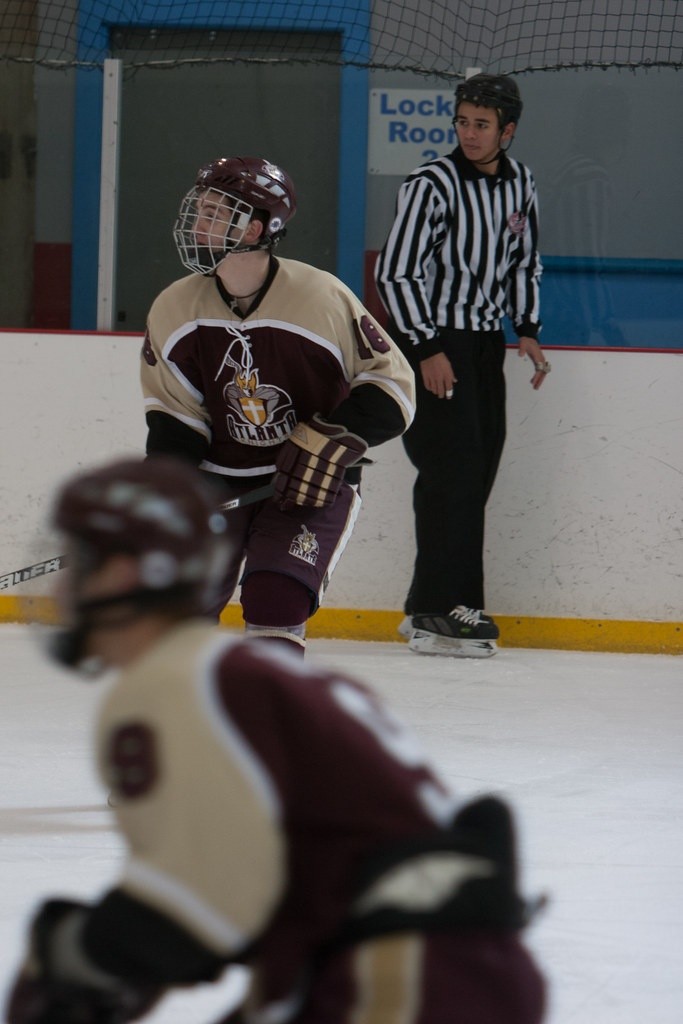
[172,157,298,275]
[456,73,522,115]
[37,459,227,675]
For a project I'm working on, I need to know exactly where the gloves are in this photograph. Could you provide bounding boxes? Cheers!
[8,899,157,1024]
[270,410,368,517]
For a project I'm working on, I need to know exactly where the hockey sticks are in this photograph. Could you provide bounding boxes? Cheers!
[0,483,276,594]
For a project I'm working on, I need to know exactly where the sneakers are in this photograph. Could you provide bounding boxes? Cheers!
[400,604,497,658]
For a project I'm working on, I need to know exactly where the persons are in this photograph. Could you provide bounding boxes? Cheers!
[371,72,551,660]
[138,154,417,661]
[1,455,549,1024]
